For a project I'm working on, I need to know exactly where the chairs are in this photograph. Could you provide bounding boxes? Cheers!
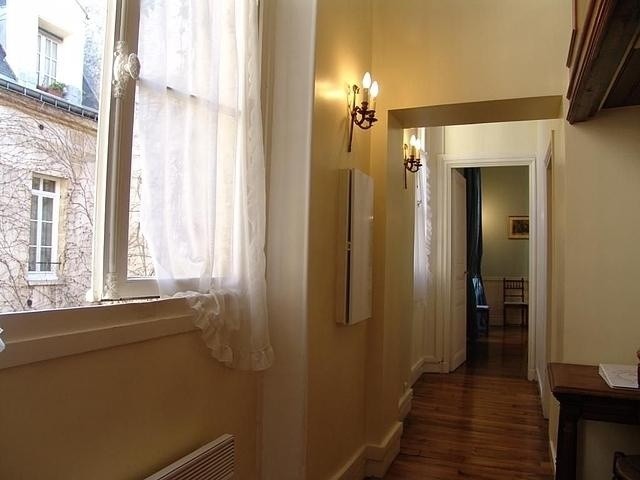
[502,277,528,331]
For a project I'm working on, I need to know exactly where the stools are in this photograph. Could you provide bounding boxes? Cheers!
[477,304,490,338]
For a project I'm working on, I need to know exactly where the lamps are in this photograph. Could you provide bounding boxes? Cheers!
[345,70,380,153]
[403,133,423,189]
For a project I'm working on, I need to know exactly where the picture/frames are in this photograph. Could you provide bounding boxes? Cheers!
[506,215,530,240]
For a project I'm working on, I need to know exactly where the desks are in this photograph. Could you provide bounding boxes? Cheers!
[546,361,640,480]
